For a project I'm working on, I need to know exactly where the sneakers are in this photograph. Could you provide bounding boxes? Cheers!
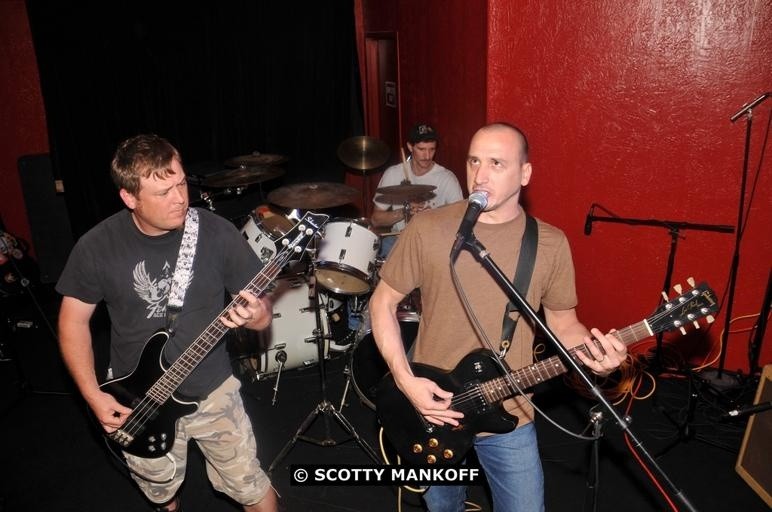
[329,329,356,351]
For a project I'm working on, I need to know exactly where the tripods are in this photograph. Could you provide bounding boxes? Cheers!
[267,247,387,489]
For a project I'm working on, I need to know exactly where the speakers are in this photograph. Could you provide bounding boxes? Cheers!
[735,364,772,511]
[15,152,77,287]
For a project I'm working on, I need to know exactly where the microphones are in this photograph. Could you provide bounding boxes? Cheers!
[585,203,597,235]
[729,88,772,124]
[722,397,771,422]
[448,192,488,259]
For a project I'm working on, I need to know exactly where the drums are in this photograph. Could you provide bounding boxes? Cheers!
[239,204,303,267]
[227,271,333,380]
[311,215,382,296]
[347,291,421,413]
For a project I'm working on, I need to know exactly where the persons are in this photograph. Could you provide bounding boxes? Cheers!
[368,121,628,512]
[371,124,464,258]
[54,133,278,512]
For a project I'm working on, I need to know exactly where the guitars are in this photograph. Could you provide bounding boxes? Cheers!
[87,210,331,459]
[375,275,722,486]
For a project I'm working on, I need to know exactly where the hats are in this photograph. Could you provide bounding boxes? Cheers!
[409,123,437,140]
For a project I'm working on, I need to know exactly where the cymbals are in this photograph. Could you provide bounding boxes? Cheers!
[222,150,285,166]
[373,183,437,205]
[201,164,284,188]
[267,181,359,211]
